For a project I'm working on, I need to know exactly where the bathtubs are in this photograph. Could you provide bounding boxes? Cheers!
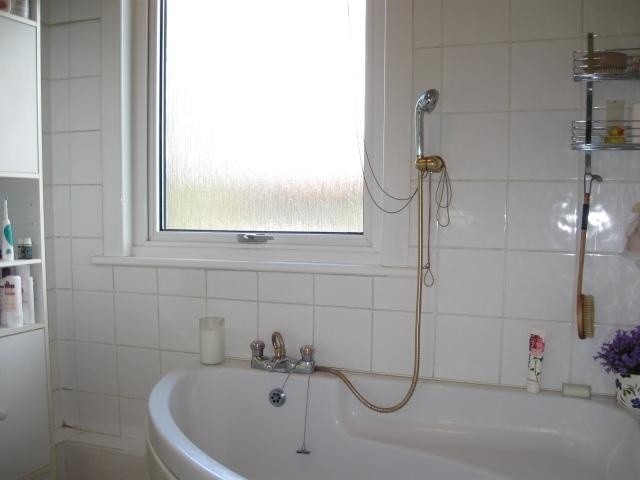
[144,356,639,479]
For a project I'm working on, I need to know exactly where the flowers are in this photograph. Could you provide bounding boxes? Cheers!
[593,326,640,378]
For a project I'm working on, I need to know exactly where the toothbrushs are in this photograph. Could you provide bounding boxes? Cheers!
[1,194,14,263]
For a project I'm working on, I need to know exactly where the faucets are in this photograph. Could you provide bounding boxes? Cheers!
[263,347,287,372]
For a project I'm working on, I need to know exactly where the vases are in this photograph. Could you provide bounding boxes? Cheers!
[614,372,640,411]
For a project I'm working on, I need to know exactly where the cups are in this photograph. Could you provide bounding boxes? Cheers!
[604,99,627,144]
[199,316,226,367]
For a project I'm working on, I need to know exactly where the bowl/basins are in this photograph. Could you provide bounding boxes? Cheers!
[584,51,626,74]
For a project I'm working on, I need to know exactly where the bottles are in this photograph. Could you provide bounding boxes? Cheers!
[16,239,33,259]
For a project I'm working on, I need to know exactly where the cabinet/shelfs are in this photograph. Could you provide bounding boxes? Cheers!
[0,0,57,480]
[569,32,640,173]
